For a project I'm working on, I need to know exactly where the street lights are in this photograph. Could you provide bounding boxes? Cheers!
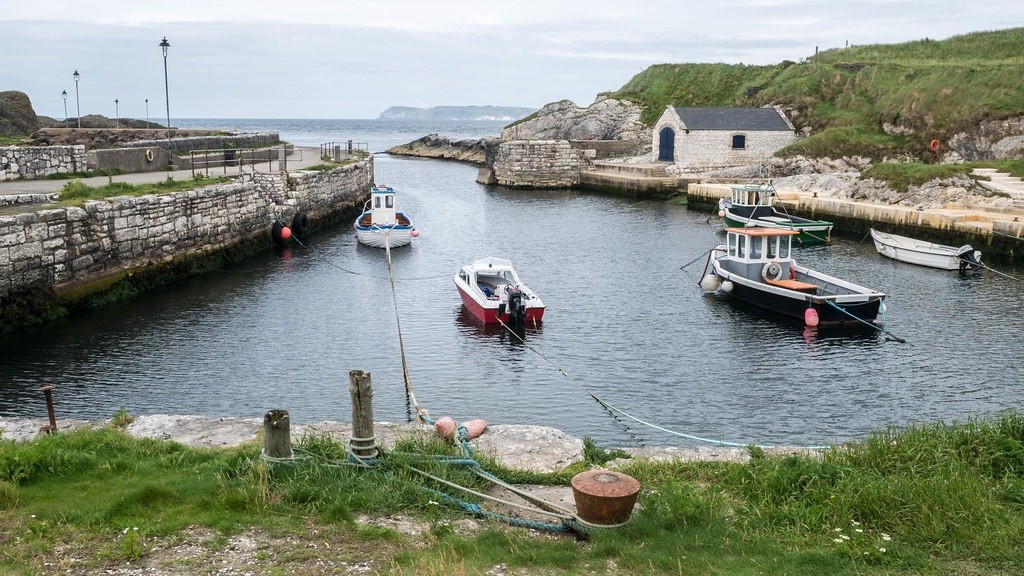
[62,90,68,127]
[159,36,171,150]
[73,71,81,128]
[145,99,149,129]
[115,99,119,129]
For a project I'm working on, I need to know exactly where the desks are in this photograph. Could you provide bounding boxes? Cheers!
[758,216,793,223]
[767,280,817,295]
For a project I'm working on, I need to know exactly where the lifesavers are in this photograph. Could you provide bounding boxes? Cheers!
[761,261,783,283]
[930,139,940,150]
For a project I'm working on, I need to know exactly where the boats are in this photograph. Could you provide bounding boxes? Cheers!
[453,256,545,324]
[354,183,413,248]
[718,152,834,245]
[869,227,983,270]
[709,227,886,328]
